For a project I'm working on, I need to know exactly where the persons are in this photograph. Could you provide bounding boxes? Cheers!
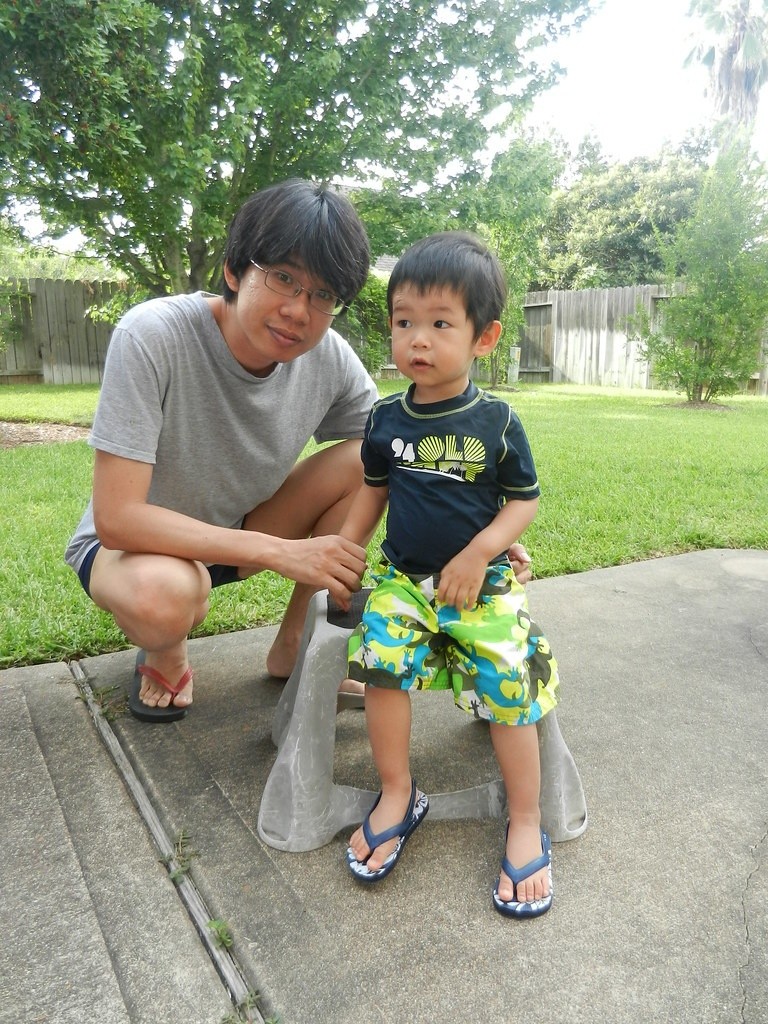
[340,231,554,920]
[64,179,532,721]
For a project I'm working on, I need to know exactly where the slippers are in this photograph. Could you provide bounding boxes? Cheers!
[129,647,196,723]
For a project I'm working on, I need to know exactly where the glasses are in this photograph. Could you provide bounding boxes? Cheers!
[248,258,351,316]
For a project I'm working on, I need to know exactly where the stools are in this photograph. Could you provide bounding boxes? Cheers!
[257,585,589,853]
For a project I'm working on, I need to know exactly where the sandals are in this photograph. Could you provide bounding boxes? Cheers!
[491,815,554,917]
[347,777,430,881]
